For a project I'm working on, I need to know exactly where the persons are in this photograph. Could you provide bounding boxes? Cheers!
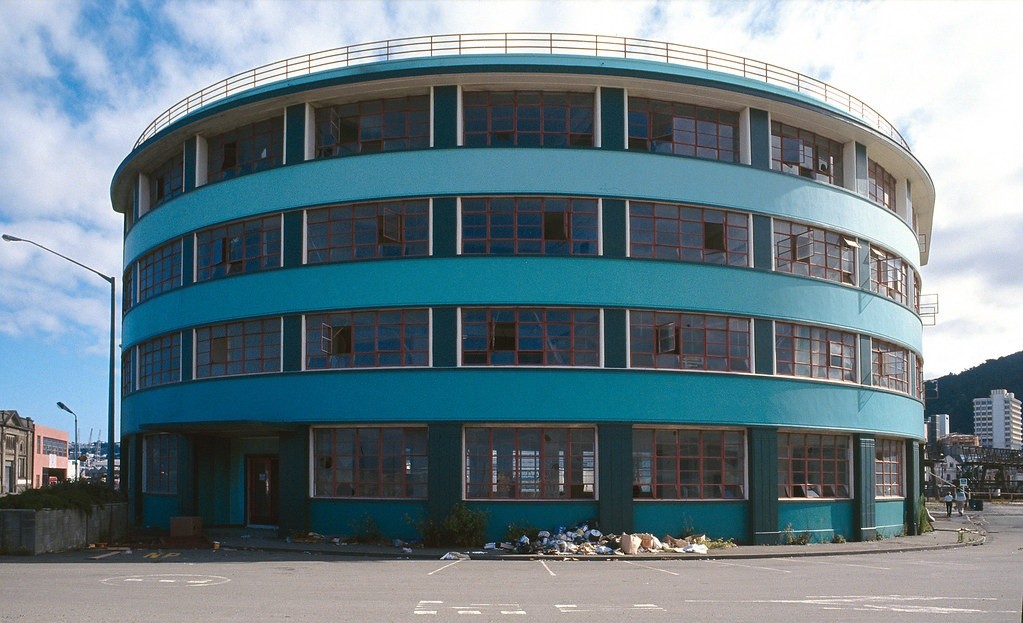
[944,492,953,517]
[956,489,966,515]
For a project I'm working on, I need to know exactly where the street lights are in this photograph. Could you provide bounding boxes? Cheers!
[55,402,78,482]
[2,233,117,495]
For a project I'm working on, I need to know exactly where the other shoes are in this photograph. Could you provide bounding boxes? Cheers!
[959,511,962,514]
[961,513,962,516]
[949,514,951,517]
[947,512,950,516]
